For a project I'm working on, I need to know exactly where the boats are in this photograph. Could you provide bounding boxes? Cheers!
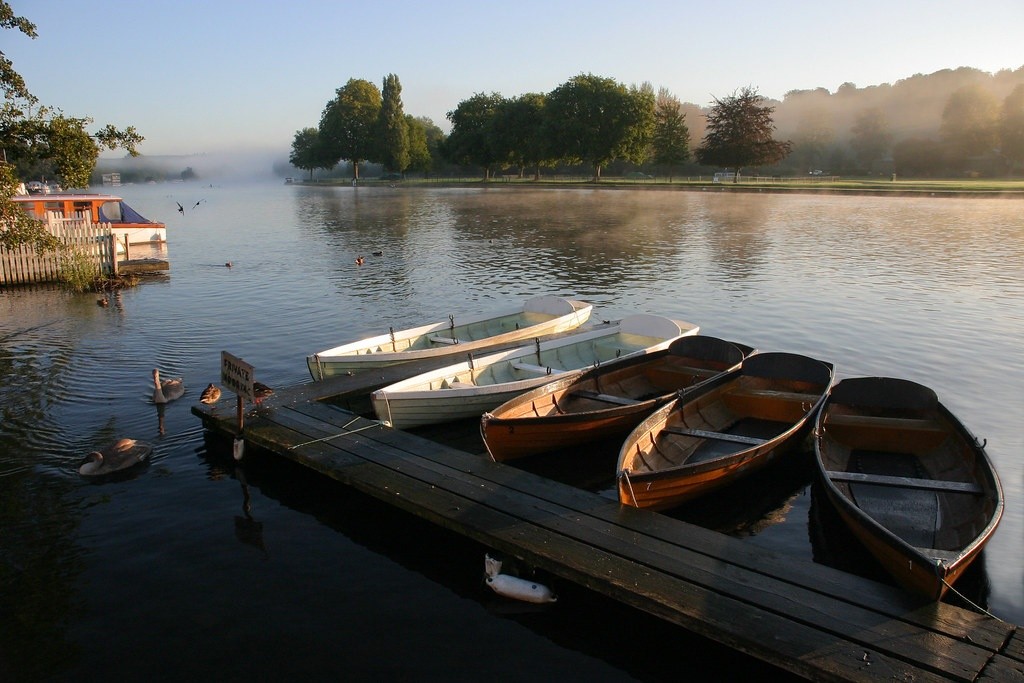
[480,335,759,466]
[616,352,835,508]
[306,298,592,383]
[370,313,700,428]
[813,376,1005,601]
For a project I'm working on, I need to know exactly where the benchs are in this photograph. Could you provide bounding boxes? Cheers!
[514,362,567,375]
[502,316,541,329]
[826,469,986,495]
[646,363,721,390]
[430,336,467,344]
[825,415,945,453]
[722,388,821,423]
[571,389,641,405]
[596,336,646,360]
[662,425,769,445]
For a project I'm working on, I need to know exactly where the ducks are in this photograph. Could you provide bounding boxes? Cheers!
[199,382,221,408]
[97,298,107,305]
[79,438,153,475]
[152,368,185,403]
[372,250,383,257]
[252,380,274,411]
[355,255,365,265]
[226,260,232,267]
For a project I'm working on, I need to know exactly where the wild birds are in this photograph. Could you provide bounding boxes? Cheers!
[175,202,185,216]
[193,198,204,210]
